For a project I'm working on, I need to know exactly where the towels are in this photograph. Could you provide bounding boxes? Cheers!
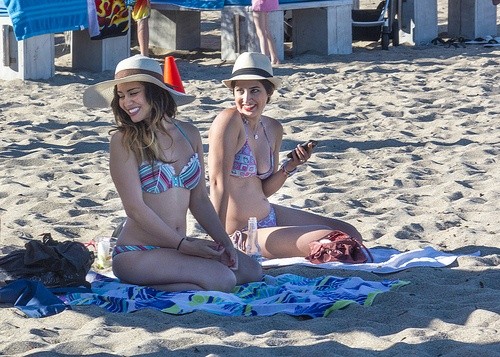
[151,0,225,12]
[0,270,411,319]
[0,0,89,41]
[88,1,100,38]
[256,244,481,274]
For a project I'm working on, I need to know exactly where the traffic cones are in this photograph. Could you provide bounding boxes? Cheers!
[163,54,186,95]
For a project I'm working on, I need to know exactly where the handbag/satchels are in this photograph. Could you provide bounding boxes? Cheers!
[305,229,374,264]
[0,233,94,288]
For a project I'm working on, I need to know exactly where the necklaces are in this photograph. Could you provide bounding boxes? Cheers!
[243,115,263,141]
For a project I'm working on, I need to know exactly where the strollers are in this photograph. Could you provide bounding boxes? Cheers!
[351,0,403,50]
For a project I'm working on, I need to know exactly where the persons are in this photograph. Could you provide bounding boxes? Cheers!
[83,54,264,293]
[252,0,281,65]
[209,51,363,258]
[130,0,154,57]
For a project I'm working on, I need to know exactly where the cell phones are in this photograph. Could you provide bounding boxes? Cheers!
[287,140,318,159]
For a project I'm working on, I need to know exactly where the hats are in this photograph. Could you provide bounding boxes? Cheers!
[83,53,196,117]
[222,51,281,90]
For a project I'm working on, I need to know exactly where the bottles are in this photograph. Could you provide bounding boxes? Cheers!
[245,216,261,264]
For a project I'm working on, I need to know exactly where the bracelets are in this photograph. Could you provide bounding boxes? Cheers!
[177,238,184,251]
